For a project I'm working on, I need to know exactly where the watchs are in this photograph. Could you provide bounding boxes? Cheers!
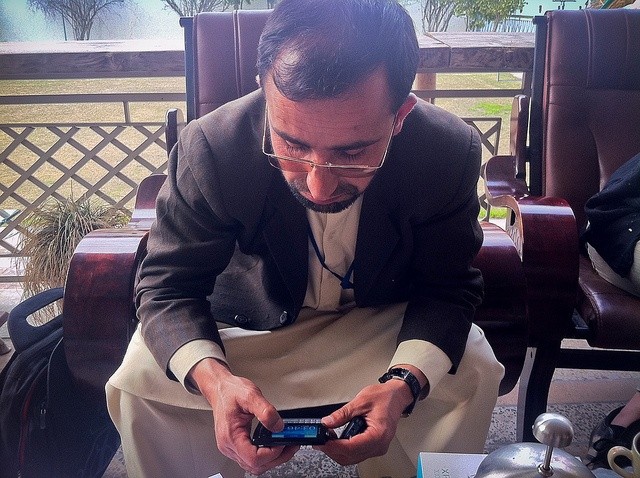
[378,366,421,418]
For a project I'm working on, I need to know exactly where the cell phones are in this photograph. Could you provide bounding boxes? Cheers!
[333,418,361,438]
[252,418,329,446]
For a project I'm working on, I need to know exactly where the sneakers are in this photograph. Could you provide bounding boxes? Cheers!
[584,403,637,472]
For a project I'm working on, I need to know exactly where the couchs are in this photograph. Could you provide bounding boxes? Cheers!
[482,8,640,458]
[61,9,530,401]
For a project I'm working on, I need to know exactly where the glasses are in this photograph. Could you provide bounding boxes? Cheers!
[261,101,399,178]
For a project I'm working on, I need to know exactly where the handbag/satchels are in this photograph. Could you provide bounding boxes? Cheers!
[0,285,122,478]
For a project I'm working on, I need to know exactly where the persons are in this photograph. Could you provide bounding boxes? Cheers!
[588,388,640,470]
[106,1,507,478]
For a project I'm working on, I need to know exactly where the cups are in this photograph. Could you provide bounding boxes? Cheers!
[607,433,640,478]
